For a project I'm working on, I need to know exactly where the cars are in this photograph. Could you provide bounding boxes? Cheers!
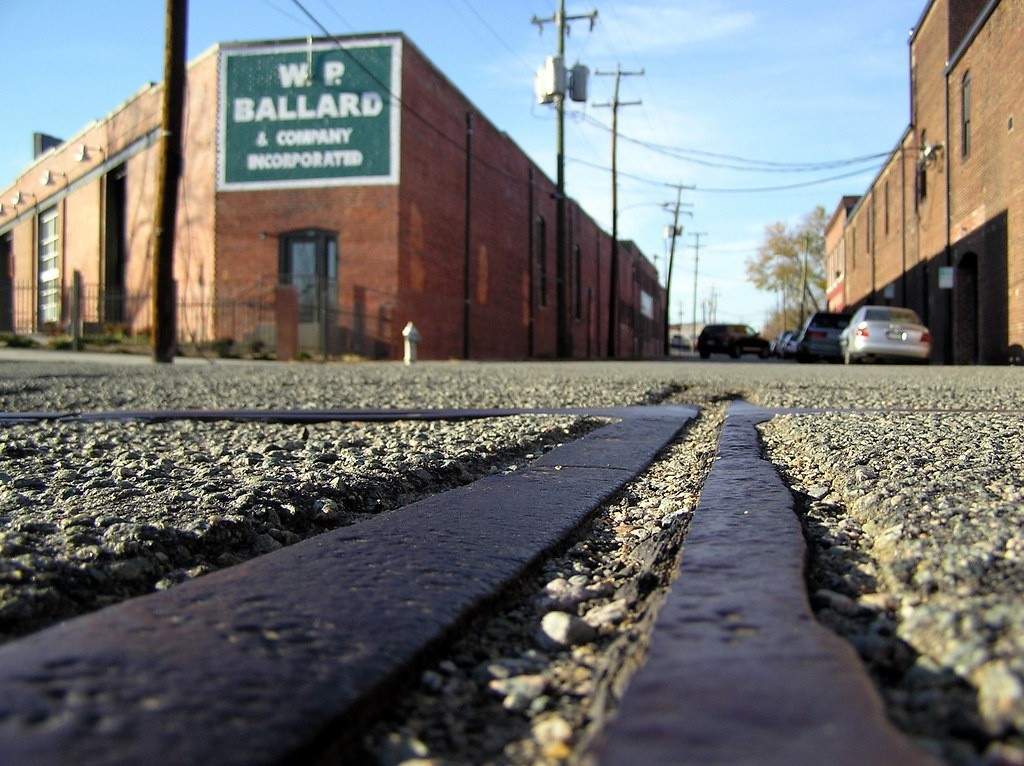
[841,305,932,364]
[771,330,797,357]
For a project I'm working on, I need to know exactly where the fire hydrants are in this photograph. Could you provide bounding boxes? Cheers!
[402,321,422,364]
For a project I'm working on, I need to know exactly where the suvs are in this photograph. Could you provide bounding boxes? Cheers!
[797,310,853,363]
[698,324,771,359]
